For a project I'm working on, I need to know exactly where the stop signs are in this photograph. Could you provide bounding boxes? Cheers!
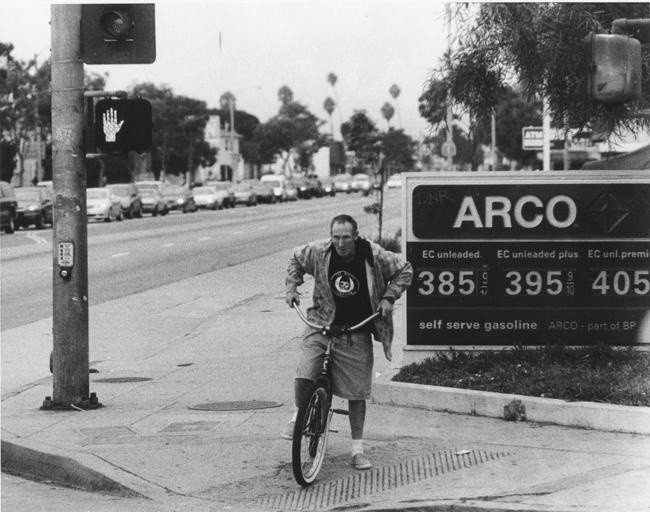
[96,98,154,152]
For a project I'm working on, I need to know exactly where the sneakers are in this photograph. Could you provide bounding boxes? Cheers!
[280,421,296,440]
[352,454,372,471]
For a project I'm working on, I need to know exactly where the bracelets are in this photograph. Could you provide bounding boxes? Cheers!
[382,295,395,305]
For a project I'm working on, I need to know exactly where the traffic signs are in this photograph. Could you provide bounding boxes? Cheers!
[405,172,650,353]
[523,127,543,151]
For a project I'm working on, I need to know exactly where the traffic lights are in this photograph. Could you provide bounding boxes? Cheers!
[100,10,137,43]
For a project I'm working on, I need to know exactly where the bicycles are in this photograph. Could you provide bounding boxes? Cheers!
[291,304,381,488]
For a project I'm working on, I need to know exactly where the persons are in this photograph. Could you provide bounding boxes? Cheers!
[281,214,416,470]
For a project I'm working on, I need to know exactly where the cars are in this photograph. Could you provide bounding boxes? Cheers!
[388,175,402,189]
